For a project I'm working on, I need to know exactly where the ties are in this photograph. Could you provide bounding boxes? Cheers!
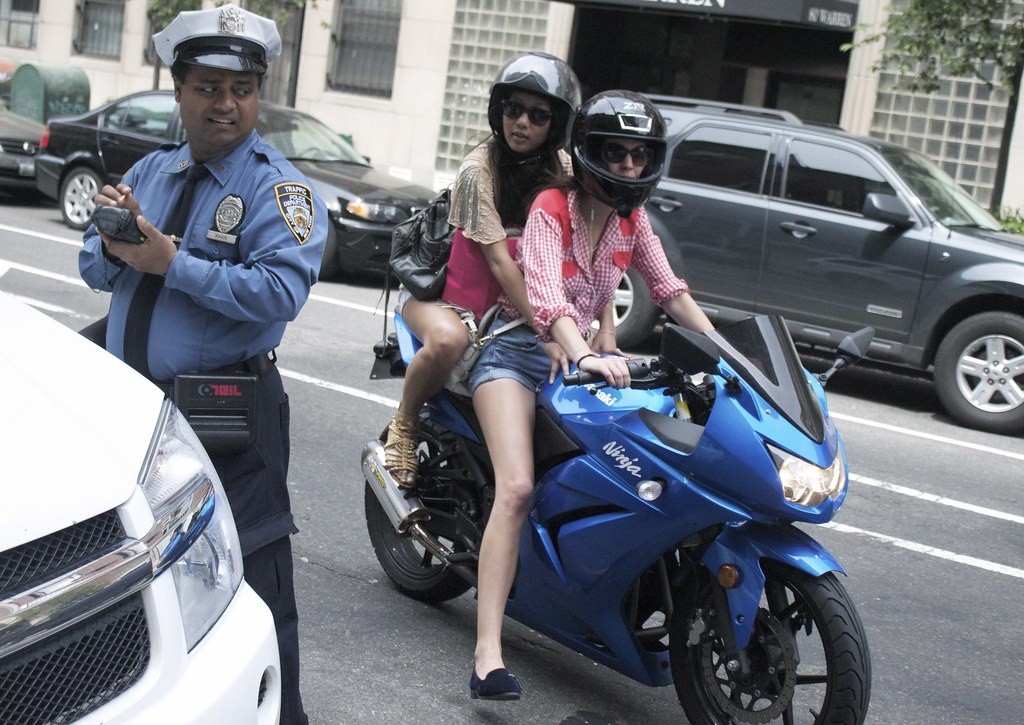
[124,163,211,385]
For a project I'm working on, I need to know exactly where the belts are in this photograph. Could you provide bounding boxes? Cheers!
[209,353,274,381]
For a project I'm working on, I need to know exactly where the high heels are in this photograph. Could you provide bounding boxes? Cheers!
[469,665,524,700]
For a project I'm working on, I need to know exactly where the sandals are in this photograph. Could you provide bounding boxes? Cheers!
[384,415,420,489]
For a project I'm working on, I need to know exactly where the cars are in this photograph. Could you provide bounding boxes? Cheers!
[36,90,452,288]
[0,99,47,205]
[0,257,284,725]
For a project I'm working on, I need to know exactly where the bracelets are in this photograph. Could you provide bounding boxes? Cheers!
[596,331,615,336]
[577,354,596,368]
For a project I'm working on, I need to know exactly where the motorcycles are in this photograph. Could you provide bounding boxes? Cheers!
[363,253,873,725]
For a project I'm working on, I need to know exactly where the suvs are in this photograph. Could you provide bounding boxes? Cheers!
[590,88,1024,436]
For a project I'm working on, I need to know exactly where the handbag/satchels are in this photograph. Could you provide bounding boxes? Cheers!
[387,189,454,301]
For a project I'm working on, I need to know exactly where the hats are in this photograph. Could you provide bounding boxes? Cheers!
[152,3,282,74]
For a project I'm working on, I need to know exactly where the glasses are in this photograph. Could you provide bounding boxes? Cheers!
[501,97,553,126]
[599,143,657,168]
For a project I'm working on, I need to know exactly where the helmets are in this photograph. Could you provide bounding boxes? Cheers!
[571,90,667,213]
[488,52,581,152]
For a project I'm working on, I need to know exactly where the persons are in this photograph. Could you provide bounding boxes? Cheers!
[383,52,582,488]
[78,3,328,725]
[467,90,718,701]
